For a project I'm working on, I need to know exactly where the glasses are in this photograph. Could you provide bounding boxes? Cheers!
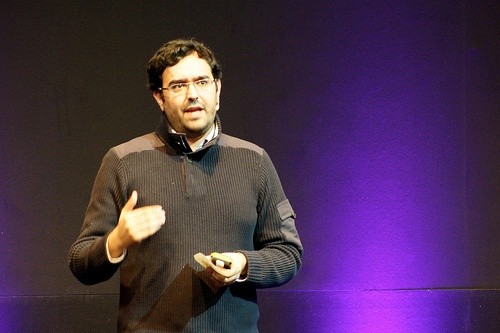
[159,79,217,93]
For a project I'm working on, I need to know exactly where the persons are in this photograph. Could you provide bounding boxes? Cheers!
[67,37,305,333]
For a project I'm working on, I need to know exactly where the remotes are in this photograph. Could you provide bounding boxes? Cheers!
[210,253,232,270]
